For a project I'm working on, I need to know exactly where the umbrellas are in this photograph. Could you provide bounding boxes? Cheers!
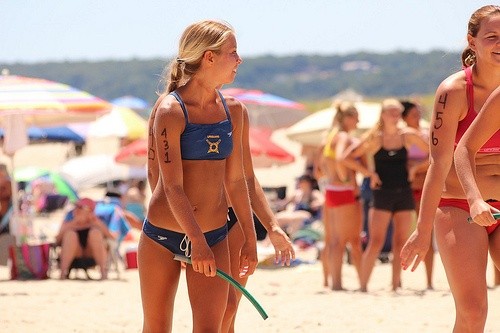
[217,88,304,127]
[115,130,295,169]
[0,75,150,203]
[285,102,430,146]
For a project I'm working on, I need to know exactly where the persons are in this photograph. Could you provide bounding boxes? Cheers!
[56,198,116,280]
[0,164,11,218]
[19,185,48,214]
[138,21,258,333]
[125,181,145,222]
[453,86,500,227]
[147,93,295,333]
[104,181,122,206]
[271,99,434,293]
[399,5,500,333]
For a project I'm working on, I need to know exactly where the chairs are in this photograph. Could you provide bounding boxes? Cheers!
[56,256,97,280]
